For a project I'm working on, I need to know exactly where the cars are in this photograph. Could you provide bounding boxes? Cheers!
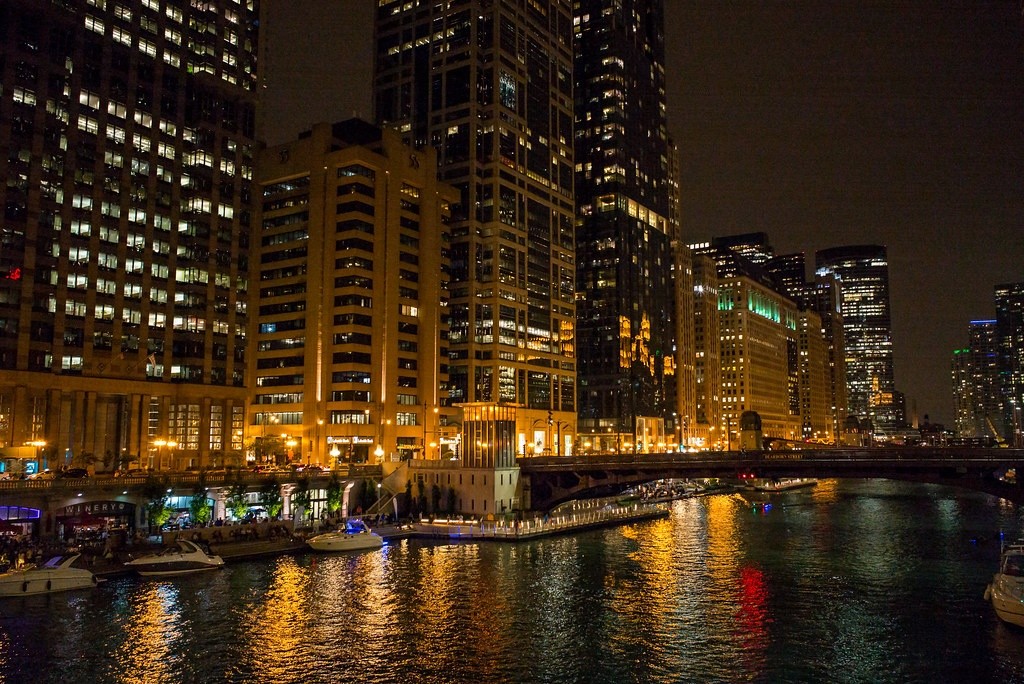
[0,472,19,487]
[260,468,283,473]
[30,469,57,481]
[288,462,322,472]
[124,467,150,478]
[62,468,90,478]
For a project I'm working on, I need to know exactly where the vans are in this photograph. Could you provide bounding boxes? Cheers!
[252,465,272,474]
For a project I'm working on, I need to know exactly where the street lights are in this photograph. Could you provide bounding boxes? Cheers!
[281,433,287,469]
[168,442,177,475]
[374,449,384,465]
[330,450,340,469]
[610,423,625,454]
[33,442,46,473]
[155,440,167,475]
[549,419,567,456]
[672,412,689,454]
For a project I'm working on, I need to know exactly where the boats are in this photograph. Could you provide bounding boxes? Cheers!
[121,538,227,578]
[304,518,384,552]
[0,550,107,599]
[981,531,1024,629]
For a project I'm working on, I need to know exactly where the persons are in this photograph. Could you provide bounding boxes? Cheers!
[479,516,484,528]
[1,528,109,572]
[135,528,146,539]
[375,513,380,528]
[408,512,413,523]
[514,521,519,532]
[429,511,433,524]
[170,513,308,543]
[419,511,422,526]
[381,512,385,526]
[543,513,548,524]
[363,515,372,529]
[321,510,325,523]
